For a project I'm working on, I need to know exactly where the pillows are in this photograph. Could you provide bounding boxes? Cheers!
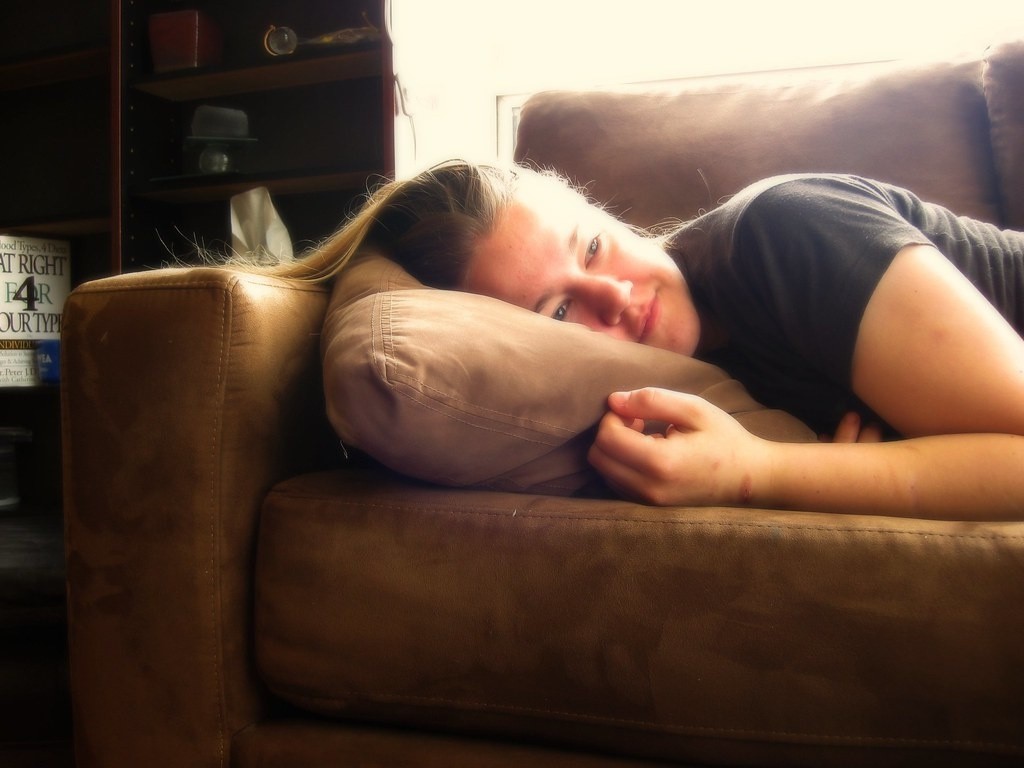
[321,236,823,495]
[983,36,1024,235]
[514,58,1001,226]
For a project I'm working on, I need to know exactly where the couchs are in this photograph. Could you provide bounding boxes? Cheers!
[59,268,1024,768]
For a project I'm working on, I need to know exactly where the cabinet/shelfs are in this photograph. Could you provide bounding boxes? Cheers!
[0,0,456,768]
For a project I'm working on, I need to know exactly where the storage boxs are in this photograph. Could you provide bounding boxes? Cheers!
[0,234,72,386]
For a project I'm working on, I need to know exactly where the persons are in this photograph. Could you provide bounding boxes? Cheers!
[188,157,1024,517]
